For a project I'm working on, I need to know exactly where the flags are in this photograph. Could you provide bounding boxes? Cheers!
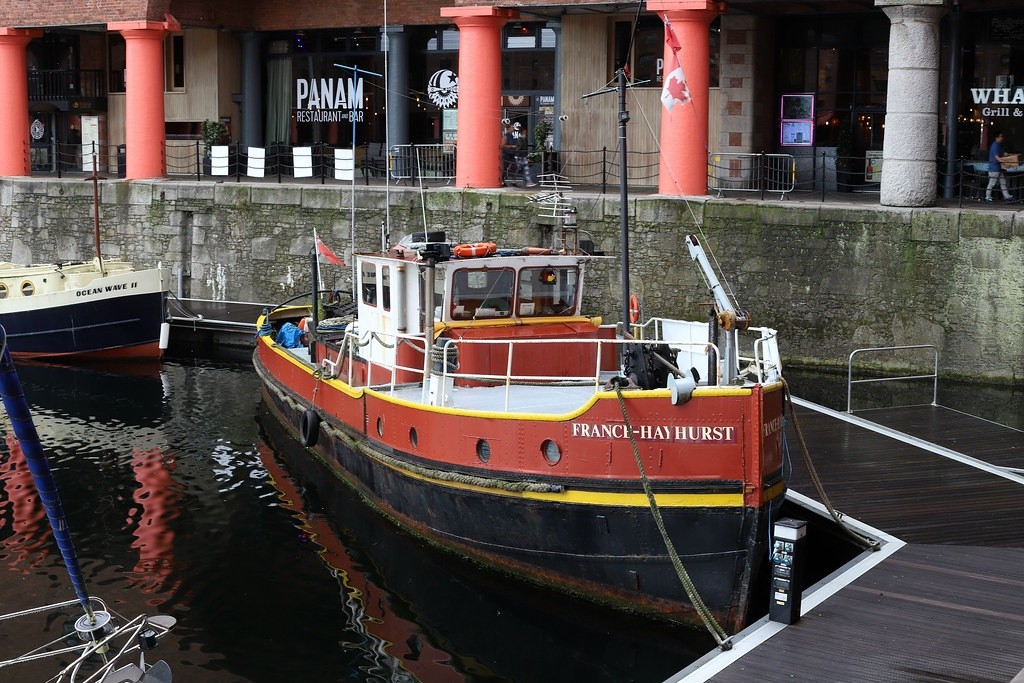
[315,230,347,269]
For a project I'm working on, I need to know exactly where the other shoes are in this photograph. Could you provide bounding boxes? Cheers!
[985,196,993,201]
[526,183,537,187]
[1005,194,1013,200]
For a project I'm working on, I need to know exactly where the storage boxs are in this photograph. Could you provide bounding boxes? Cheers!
[1001,153,1023,168]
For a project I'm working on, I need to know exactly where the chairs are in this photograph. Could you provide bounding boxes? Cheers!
[361,143,381,178]
[374,144,387,178]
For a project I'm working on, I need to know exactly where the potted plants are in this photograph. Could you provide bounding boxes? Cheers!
[526,120,560,185]
[200,119,229,175]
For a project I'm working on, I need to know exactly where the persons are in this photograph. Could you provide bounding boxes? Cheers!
[984,131,1017,203]
[500,123,519,188]
[512,127,538,187]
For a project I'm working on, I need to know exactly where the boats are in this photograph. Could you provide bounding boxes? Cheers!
[252,68,791,647]
[0,139,170,367]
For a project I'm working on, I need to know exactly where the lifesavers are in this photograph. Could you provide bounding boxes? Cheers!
[453,242,497,256]
[629,294,639,324]
